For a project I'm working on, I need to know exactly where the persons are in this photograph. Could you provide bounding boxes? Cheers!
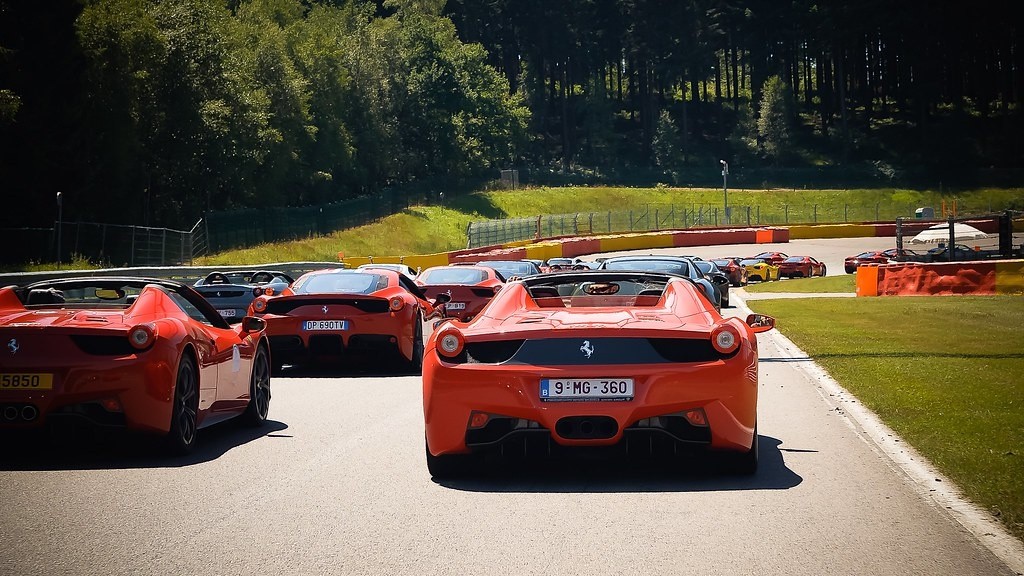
[588,284,616,294]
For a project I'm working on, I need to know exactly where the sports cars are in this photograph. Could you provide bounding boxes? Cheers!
[182,270,296,323]
[242,267,450,372]
[346,223,971,321]
[420,272,778,476]
[1,267,271,454]
